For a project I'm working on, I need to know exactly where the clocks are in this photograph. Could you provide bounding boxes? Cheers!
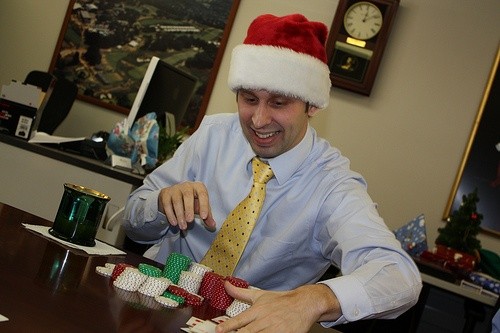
[324,0,401,99]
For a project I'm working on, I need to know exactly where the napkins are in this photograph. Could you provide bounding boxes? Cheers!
[20,221,128,259]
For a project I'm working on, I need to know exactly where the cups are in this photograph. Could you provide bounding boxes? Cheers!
[35,242,89,295]
[48,183,111,247]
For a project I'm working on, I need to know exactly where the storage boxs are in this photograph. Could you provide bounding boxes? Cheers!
[0,79,46,141]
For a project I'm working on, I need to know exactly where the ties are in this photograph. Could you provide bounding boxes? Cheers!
[199,156,275,278]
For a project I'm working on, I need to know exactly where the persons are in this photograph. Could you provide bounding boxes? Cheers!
[122,12,422,333]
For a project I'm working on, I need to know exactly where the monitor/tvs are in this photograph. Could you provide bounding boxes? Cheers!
[122,56,199,134]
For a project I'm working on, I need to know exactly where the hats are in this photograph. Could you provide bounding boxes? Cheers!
[228,13,331,110]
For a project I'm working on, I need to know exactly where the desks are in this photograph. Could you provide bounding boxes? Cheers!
[0,201,227,333]
[410,255,500,333]
[0,128,147,188]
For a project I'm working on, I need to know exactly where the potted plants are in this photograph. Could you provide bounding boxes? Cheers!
[434,185,484,272]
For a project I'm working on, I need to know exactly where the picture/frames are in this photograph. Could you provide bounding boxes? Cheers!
[439,46,500,240]
[48,0,242,135]
